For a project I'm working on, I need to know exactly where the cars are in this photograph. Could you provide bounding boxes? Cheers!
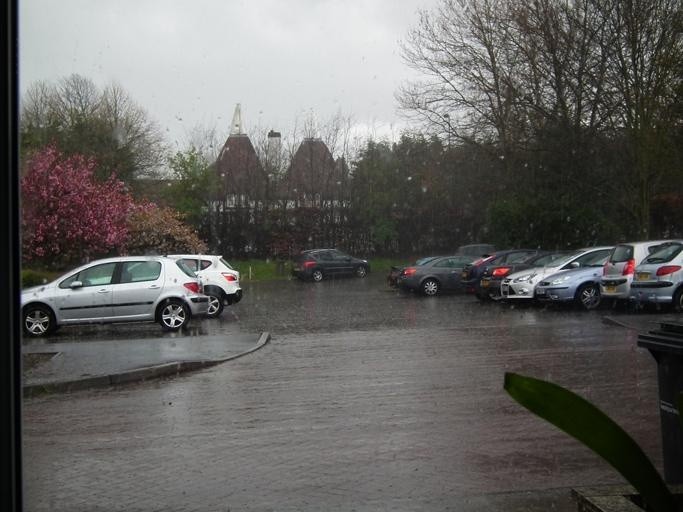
[20,256,210,338]
[501,246,615,303]
[462,250,552,302]
[630,244,683,313]
[399,256,485,296]
[89,255,241,318]
[600,240,683,301]
[534,247,617,311]
[481,252,571,302]
[388,257,445,291]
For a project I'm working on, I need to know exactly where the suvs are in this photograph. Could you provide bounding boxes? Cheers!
[292,249,370,280]
[455,243,497,261]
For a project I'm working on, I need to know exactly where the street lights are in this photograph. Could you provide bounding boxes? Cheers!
[443,113,450,150]
